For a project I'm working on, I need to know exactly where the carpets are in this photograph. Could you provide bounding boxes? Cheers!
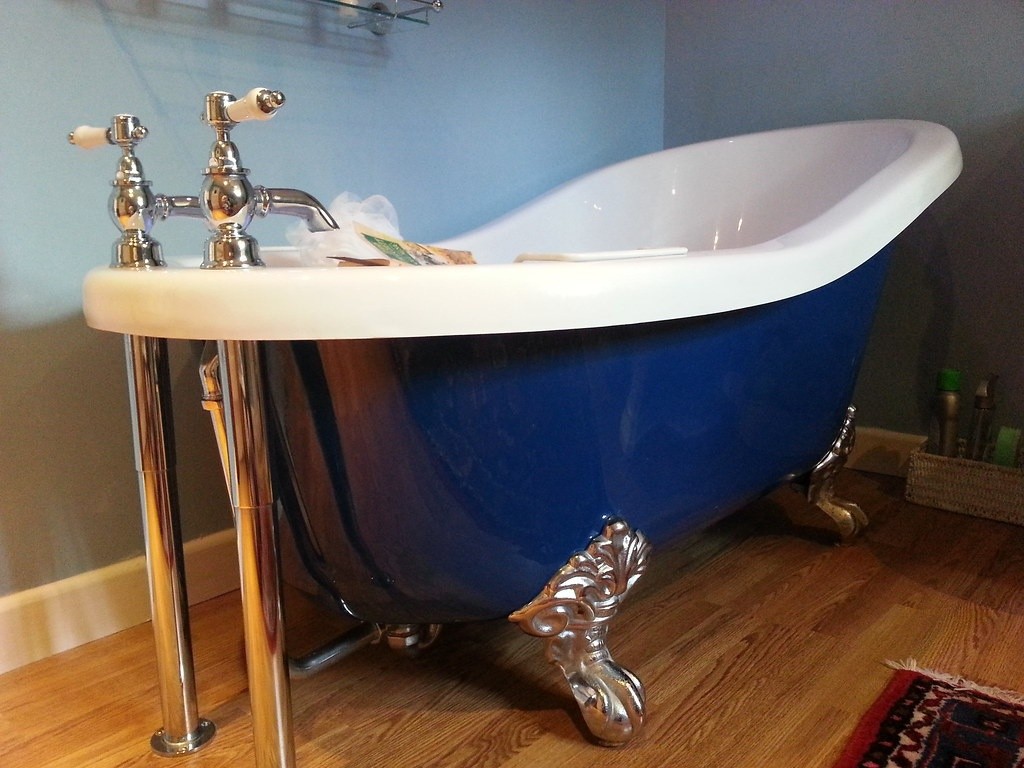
[827,656,1024,768]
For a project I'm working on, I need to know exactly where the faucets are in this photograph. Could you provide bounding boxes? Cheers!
[199,86,341,270]
[67,112,204,269]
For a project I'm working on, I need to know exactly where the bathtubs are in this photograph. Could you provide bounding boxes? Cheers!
[82,121,962,768]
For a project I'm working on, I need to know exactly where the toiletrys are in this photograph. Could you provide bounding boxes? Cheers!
[927,367,1000,462]
